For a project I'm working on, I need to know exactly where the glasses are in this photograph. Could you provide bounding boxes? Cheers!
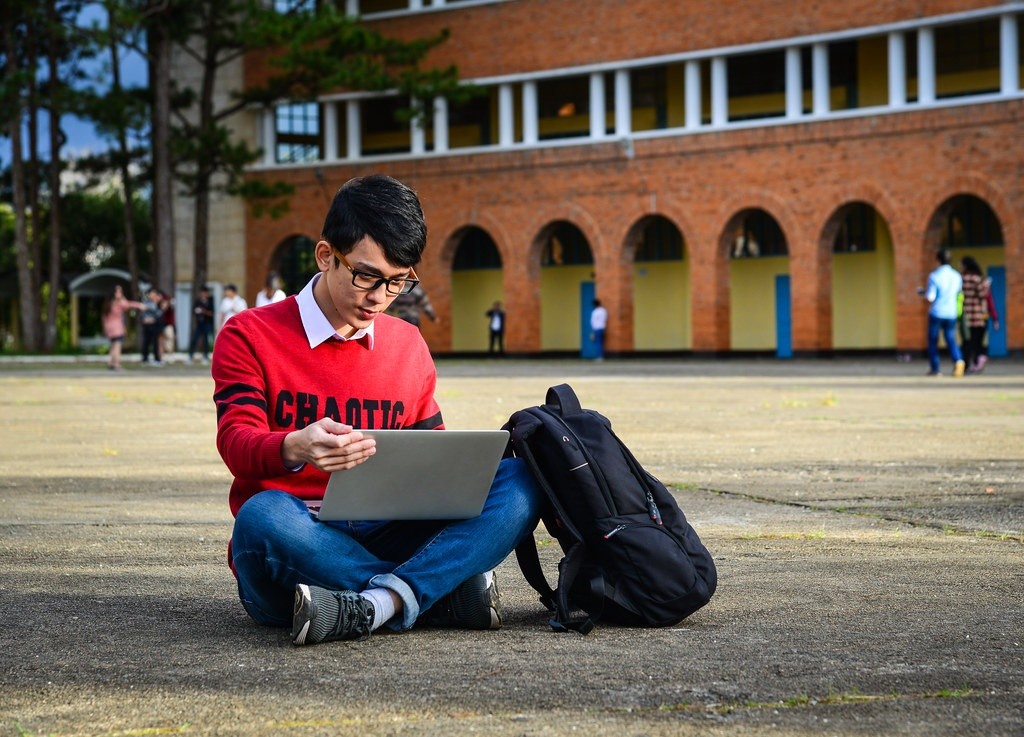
[319,233,420,296]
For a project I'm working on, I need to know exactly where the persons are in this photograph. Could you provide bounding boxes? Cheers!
[486,301,506,353]
[212,173,542,648]
[102,274,288,370]
[919,250,999,378]
[589,299,607,362]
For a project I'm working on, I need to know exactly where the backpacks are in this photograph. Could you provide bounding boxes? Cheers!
[499,381,719,636]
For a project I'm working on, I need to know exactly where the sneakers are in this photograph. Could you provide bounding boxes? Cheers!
[416,570,503,631]
[291,581,376,648]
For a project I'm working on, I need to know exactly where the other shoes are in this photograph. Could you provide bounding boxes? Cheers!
[201,357,210,366]
[140,359,149,367]
[925,371,943,378]
[188,357,194,364]
[950,360,966,379]
[109,364,123,371]
[968,354,990,374]
[152,360,164,367]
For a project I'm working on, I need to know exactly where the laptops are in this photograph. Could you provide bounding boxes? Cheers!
[304,429,511,522]
